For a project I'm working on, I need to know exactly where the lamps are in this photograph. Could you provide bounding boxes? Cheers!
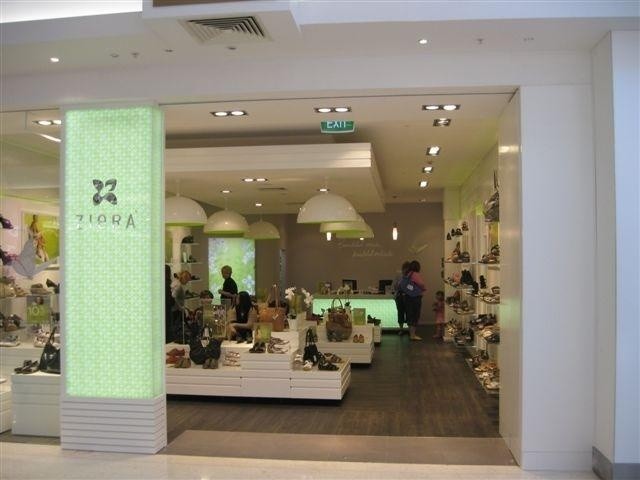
[165,181,208,228]
[295,176,360,226]
[336,225,375,241]
[242,207,281,241]
[318,213,367,235]
[203,194,252,236]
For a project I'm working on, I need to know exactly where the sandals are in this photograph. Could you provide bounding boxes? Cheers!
[1,313,22,331]
[444,318,472,346]
[257,342,266,353]
[468,349,500,390]
[303,360,312,370]
[469,312,501,343]
[318,359,339,371]
[445,289,470,315]
[472,274,500,303]
[24,360,40,374]
[324,352,341,362]
[14,360,32,373]
[295,352,304,362]
[249,342,259,353]
[4,274,29,298]
[480,243,499,265]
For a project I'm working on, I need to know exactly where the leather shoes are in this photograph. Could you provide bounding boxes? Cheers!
[33,326,60,350]
[272,338,289,344]
[447,243,471,265]
[446,222,468,239]
[1,333,20,347]
[202,358,210,369]
[269,342,288,355]
[367,314,381,325]
[353,335,359,343]
[210,360,218,370]
[358,334,364,343]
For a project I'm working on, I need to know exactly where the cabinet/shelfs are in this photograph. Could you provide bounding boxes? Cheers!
[1,383,12,435]
[445,192,499,398]
[0,333,56,367]
[361,321,382,345]
[12,367,64,440]
[160,333,351,405]
[273,318,372,366]
[172,242,204,312]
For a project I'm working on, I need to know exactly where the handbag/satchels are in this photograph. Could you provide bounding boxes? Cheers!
[39,328,62,373]
[399,274,423,297]
[257,283,283,332]
[187,325,222,364]
[327,296,351,339]
[303,330,320,365]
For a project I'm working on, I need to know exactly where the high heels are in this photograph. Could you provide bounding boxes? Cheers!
[46,279,60,293]
[0,215,13,229]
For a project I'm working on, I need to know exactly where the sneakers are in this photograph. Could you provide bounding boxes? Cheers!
[166,355,179,364]
[443,269,473,288]
[166,347,185,357]
[172,358,182,367]
[182,358,191,368]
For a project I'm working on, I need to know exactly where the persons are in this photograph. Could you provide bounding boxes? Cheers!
[28,215,50,262]
[165,263,257,363]
[391,260,428,341]
[432,291,445,339]
[31,297,45,306]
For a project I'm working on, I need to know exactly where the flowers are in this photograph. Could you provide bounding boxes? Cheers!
[285,285,313,316]
